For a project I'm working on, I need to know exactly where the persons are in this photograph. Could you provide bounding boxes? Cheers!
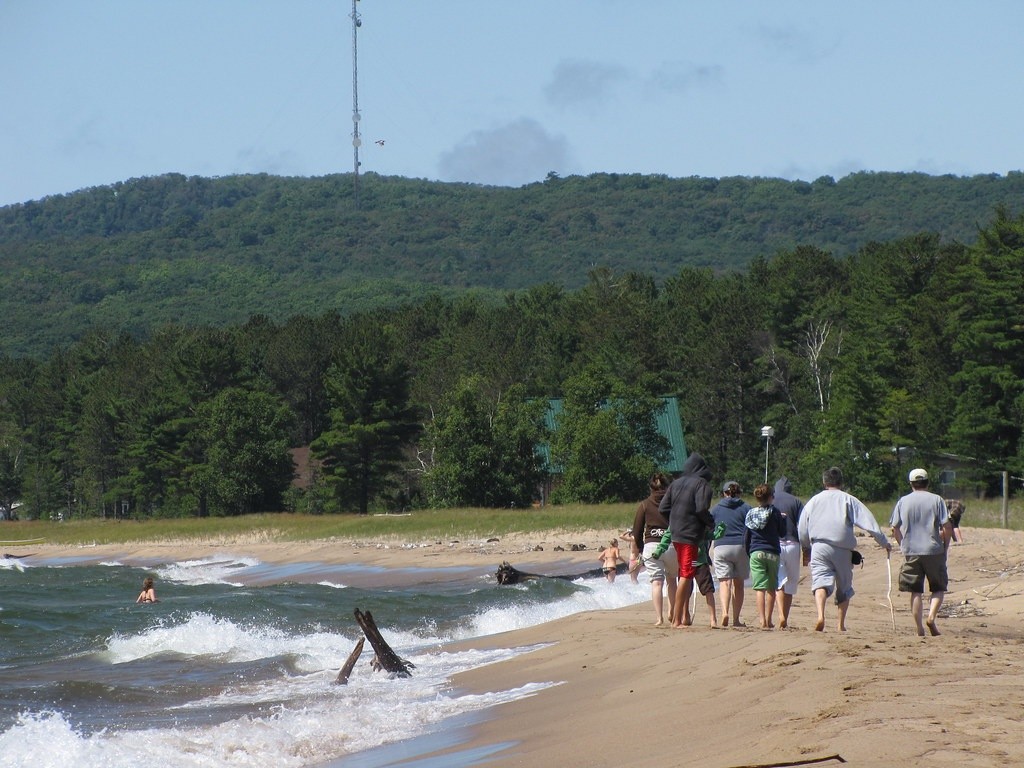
[890,469,958,636]
[619,528,642,584]
[659,452,715,627]
[742,483,787,628]
[771,475,805,627]
[651,521,727,628]
[797,467,892,631]
[136,577,160,603]
[633,472,679,625]
[598,538,627,583]
[710,481,753,627]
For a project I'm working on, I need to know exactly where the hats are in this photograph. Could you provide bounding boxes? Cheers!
[909,469,928,481]
[723,481,743,492]
